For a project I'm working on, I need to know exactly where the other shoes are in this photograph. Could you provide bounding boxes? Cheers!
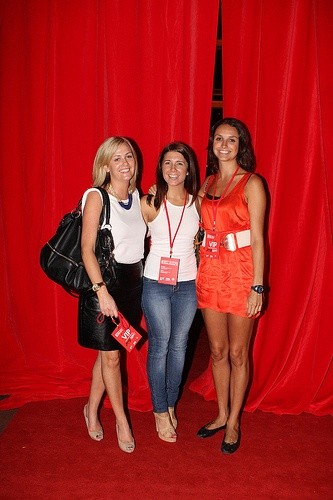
[197,419,241,454]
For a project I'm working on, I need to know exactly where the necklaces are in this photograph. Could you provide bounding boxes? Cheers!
[107,182,133,210]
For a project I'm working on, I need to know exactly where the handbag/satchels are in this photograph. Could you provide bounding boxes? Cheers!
[39,186,119,295]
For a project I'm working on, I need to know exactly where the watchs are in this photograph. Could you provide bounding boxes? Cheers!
[251,285,265,294]
[92,281,106,292]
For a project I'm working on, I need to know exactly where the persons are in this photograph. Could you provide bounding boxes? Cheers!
[140,141,201,443]
[148,118,267,454]
[77,136,148,454]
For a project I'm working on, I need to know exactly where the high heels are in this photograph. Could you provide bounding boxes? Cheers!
[153,407,178,442]
[83,403,135,454]
